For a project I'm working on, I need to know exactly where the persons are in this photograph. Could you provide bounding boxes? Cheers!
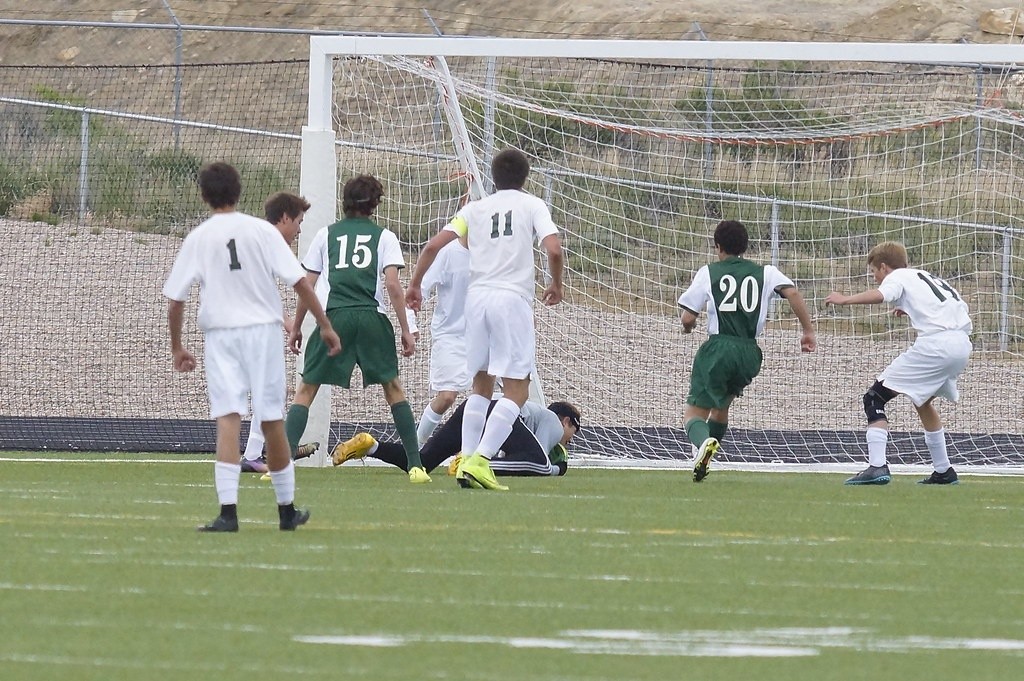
[260,173,433,484]
[241,192,311,473]
[405,150,563,490]
[825,241,973,486]
[163,163,341,532]
[406,231,505,458]
[678,220,816,483]
[333,393,580,476]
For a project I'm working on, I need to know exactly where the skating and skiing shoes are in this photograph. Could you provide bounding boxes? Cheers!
[456,453,509,491]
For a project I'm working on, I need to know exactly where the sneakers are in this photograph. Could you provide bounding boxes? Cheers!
[333,433,375,467]
[260,475,271,481]
[197,516,239,532]
[279,509,310,531]
[691,437,720,482]
[917,467,960,486]
[241,455,268,472]
[408,467,433,483]
[844,465,891,485]
[449,455,463,475]
